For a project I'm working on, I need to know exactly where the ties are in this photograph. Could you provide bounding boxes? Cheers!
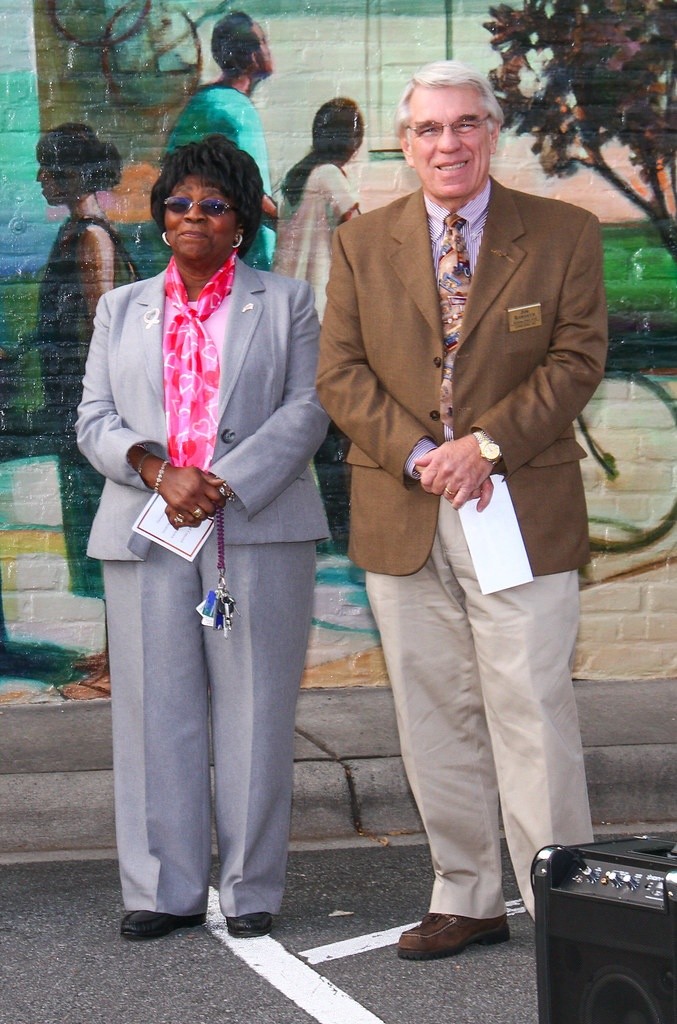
[436,214,472,431]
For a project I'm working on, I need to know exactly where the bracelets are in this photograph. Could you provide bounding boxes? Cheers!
[216,476,236,503]
[138,452,151,473]
[154,460,170,493]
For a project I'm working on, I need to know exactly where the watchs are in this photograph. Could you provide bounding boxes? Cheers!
[473,430,503,466]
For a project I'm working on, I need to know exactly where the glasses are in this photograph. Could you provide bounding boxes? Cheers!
[164,195,238,217]
[410,114,491,137]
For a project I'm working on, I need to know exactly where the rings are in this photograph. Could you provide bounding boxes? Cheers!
[445,488,456,495]
[174,514,184,523]
[193,508,203,519]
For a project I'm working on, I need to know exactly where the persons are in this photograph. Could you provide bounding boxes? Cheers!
[314,60,608,960]
[74,134,331,938]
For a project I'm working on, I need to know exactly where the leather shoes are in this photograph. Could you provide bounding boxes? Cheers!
[398,912,510,960]
[226,912,272,938]
[121,910,206,940]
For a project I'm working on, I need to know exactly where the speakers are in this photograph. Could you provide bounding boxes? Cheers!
[535,831,677,1024]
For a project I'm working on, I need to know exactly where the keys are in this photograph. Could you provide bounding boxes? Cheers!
[216,591,240,639]
